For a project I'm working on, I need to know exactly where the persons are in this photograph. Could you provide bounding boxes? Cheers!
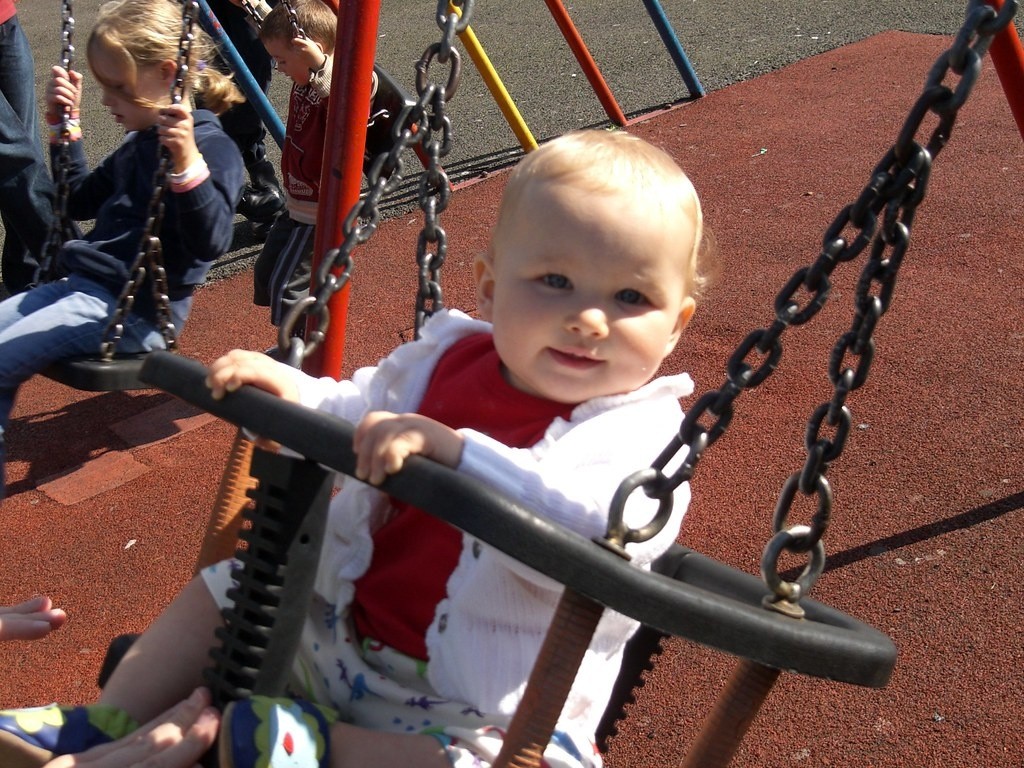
[0,131,703,767]
[179,0,285,223]
[0,597,222,768]
[230,0,335,337]
[0,0,243,436]
[0,0,84,293]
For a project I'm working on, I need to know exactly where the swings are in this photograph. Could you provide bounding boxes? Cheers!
[97,0,1021,768]
[26,0,202,393]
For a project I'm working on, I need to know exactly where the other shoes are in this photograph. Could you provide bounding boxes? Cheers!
[0,702,142,768]
[219,695,340,768]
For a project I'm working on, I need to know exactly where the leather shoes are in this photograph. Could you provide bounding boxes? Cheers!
[235,185,285,216]
